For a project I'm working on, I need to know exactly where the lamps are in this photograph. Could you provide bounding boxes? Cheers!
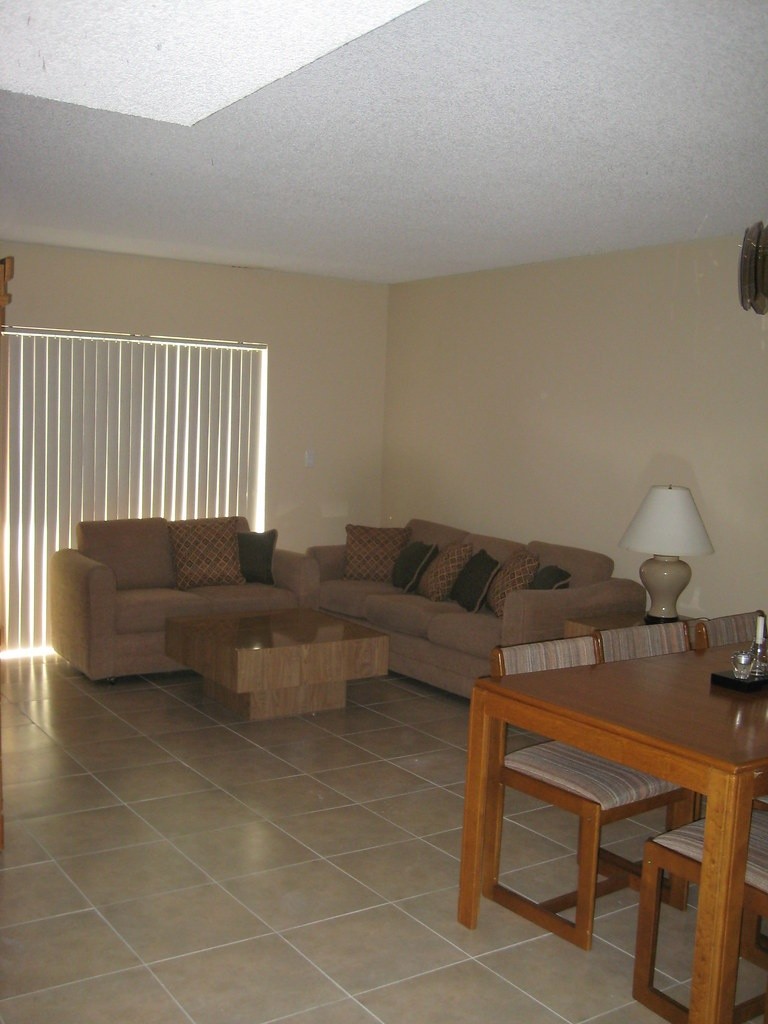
[618,486,717,625]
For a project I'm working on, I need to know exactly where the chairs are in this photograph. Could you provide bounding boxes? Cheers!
[631,797,767,1024]
[695,609,767,648]
[594,622,696,887]
[489,635,701,950]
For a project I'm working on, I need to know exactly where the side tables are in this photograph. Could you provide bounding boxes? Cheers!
[565,608,698,636]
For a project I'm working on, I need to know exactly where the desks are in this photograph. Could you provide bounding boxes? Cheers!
[456,638,768,1023]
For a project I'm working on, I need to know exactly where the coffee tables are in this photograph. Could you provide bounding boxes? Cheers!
[165,608,388,719]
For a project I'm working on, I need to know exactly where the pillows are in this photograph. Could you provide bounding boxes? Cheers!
[237,528,278,586]
[167,517,246,592]
[343,524,572,617]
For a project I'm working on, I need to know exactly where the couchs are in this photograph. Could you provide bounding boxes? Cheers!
[46,516,319,684]
[308,518,646,699]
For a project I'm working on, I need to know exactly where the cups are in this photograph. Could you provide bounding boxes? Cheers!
[749,637,768,677]
[730,651,755,679]
